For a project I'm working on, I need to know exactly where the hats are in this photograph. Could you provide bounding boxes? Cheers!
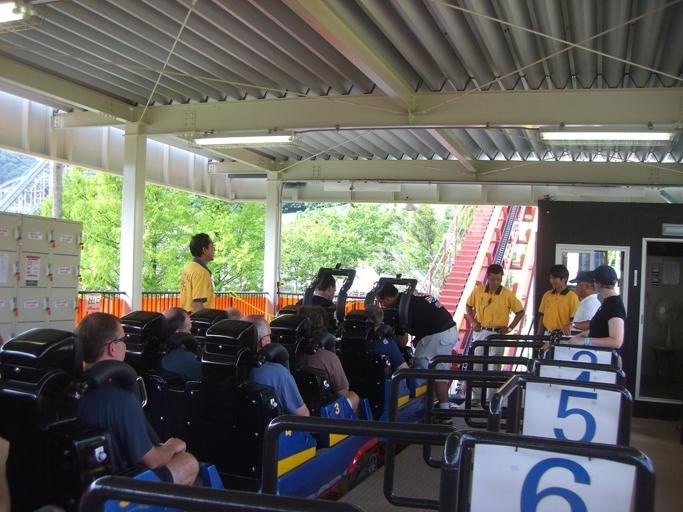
[589,265,617,285]
[569,271,594,284]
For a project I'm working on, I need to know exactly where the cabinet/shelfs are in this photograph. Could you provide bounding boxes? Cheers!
[0,212,83,349]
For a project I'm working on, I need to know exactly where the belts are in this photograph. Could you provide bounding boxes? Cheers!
[545,328,551,333]
[481,327,498,332]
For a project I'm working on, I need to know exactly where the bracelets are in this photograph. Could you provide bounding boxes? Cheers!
[507,327,512,331]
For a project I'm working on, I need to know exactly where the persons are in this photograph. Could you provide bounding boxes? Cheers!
[0,437,64,512]
[73,312,200,487]
[534,265,626,351]
[365,304,409,371]
[375,282,458,425]
[241,314,310,417]
[179,233,215,316]
[295,306,360,414]
[226,308,243,321]
[466,264,525,409]
[161,307,202,385]
[312,270,335,307]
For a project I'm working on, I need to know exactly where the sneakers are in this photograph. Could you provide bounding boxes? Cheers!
[471,398,491,410]
[427,415,454,425]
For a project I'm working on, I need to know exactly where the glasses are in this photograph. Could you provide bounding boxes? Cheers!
[107,333,130,345]
